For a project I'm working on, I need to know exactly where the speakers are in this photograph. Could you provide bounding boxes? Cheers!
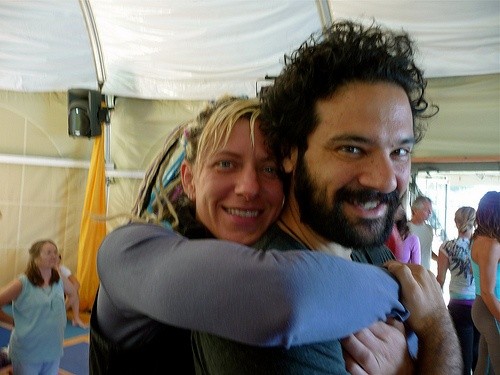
[67,88,102,138]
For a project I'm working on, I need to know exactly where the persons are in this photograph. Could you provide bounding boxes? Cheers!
[435,206,480,375]
[386,202,420,268]
[467,190,500,375]
[406,196,434,278]
[0,239,67,375]
[181,21,463,375]
[87,94,409,375]
[56,254,88,329]
